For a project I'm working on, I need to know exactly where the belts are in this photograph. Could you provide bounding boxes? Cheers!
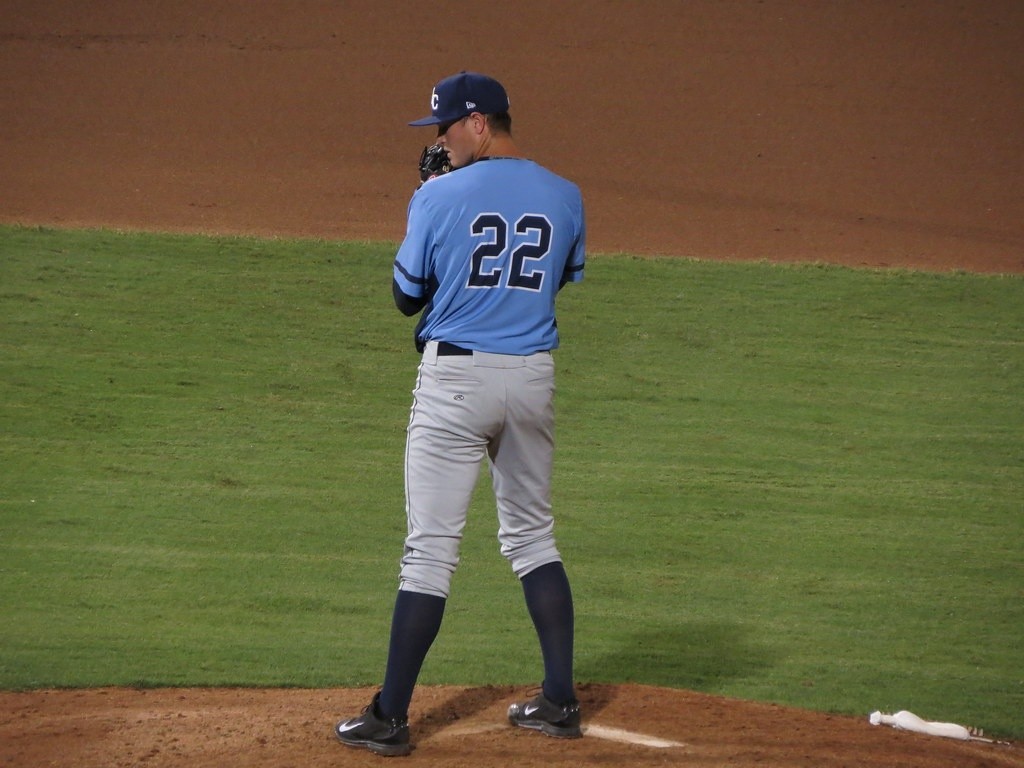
[437,341,550,355]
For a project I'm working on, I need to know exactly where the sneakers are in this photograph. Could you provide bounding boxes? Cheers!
[334,691,410,756]
[507,684,581,739]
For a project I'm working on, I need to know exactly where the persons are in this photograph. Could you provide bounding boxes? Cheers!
[335,71,586,757]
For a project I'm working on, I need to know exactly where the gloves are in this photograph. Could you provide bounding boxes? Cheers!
[419,144,455,183]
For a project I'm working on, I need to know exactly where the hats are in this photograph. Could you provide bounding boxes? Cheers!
[408,71,510,126]
